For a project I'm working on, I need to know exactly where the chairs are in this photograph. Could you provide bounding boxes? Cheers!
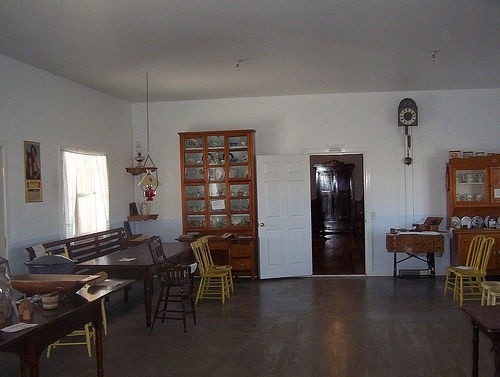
[190,240,230,305]
[147,234,198,335]
[45,245,96,359]
[453,236,495,307]
[442,234,487,302]
[124,220,141,239]
[197,237,234,293]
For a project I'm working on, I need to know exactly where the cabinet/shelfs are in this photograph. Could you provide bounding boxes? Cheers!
[177,128,259,282]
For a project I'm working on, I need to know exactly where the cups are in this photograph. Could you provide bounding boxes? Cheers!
[18,299,34,324]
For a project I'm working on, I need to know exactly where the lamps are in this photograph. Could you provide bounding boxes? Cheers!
[136,73,163,202]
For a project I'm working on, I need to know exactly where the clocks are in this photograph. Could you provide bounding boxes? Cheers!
[396,97,419,166]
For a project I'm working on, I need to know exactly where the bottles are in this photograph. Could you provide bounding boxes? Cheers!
[0,266,14,324]
[88,272,108,284]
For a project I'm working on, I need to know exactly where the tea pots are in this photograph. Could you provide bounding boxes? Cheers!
[137,201,151,216]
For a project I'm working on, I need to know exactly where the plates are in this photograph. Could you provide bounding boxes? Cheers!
[187,139,198,147]
[209,136,223,146]
[451,216,500,229]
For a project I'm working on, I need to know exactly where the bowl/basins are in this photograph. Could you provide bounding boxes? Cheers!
[230,143,237,146]
[230,158,238,163]
[221,160,225,164]
[466,194,482,201]
[197,161,203,165]
[241,155,248,163]
[456,178,483,183]
[42,293,59,310]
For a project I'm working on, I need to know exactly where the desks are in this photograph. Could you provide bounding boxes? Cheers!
[0,278,137,377]
[75,240,193,328]
[384,232,445,287]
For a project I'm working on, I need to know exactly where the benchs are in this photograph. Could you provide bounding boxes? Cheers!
[24,226,131,303]
[460,300,500,377]
